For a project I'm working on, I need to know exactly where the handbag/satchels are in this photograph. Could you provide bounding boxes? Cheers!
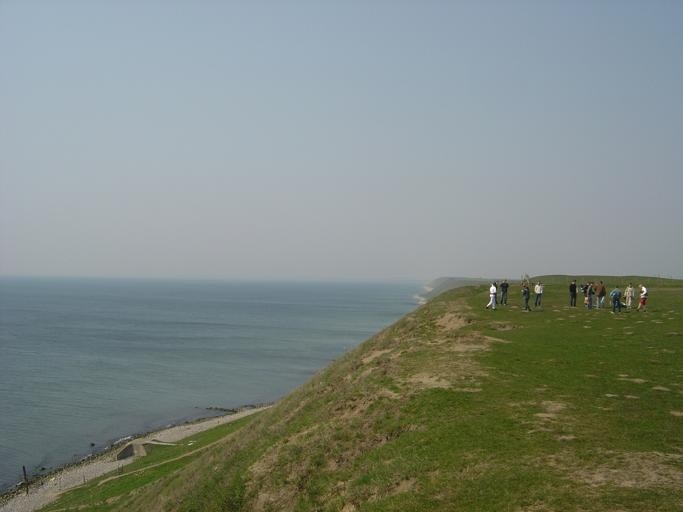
[601,296,605,303]
[584,297,588,303]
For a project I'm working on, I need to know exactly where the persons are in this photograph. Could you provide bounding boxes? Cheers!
[533,281,543,308]
[567,279,577,307]
[520,286,531,311]
[485,282,497,311]
[498,279,509,305]
[581,281,648,313]
